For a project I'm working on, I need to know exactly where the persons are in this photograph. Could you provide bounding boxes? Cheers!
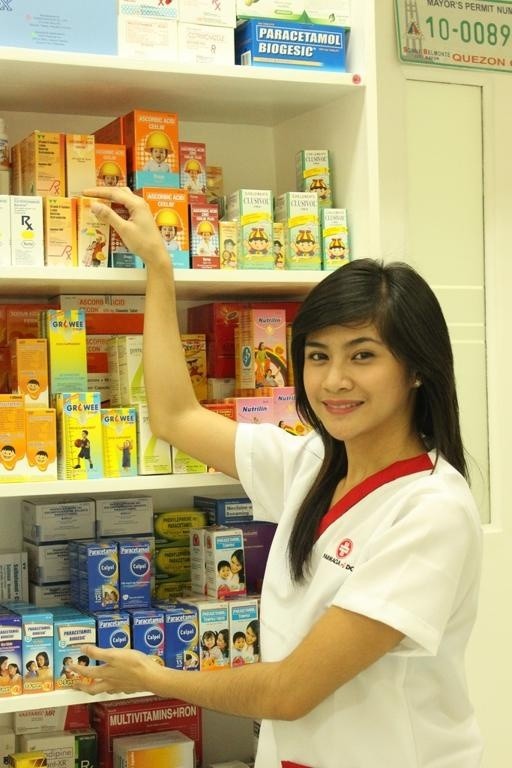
[64,183,487,768]
[0,549,260,696]
[87,130,349,266]
[253,340,298,437]
[0,380,135,477]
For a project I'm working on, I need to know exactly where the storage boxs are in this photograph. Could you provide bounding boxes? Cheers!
[235,19,347,74]
[0,1,378,768]
